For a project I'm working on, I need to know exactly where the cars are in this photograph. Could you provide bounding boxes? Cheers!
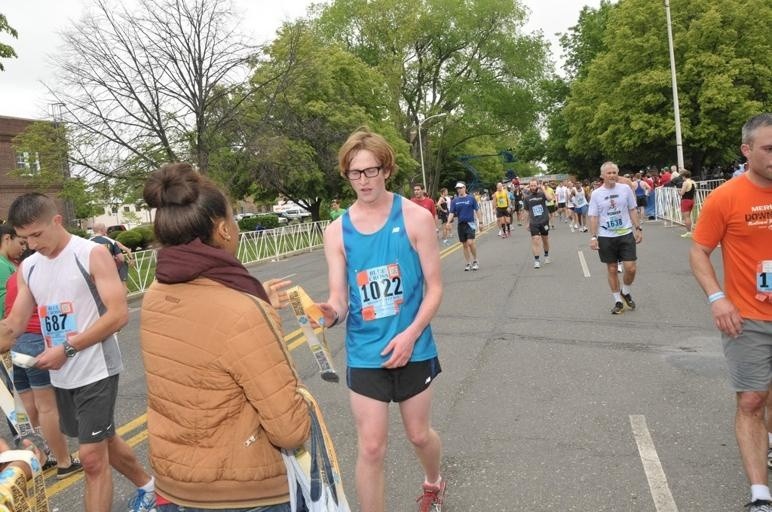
[89,206,310,246]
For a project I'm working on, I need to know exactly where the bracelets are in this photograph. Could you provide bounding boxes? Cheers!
[706,290,726,304]
[327,311,340,329]
[130,256,134,259]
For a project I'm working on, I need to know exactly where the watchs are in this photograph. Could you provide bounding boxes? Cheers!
[62,340,78,358]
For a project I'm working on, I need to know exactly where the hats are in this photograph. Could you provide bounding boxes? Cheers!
[454,183,466,188]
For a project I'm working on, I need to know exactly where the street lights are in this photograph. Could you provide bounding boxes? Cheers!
[417,111,448,193]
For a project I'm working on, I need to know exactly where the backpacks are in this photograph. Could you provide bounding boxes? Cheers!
[282,386,355,512]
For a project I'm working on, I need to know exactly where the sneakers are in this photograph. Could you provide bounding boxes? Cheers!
[416,474,447,512]
[680,232,693,238]
[748,499,772,511]
[618,264,624,273]
[471,261,480,270]
[464,264,471,271]
[498,225,514,239]
[534,261,541,268]
[543,256,551,264]
[620,289,635,311]
[56,458,84,479]
[569,222,588,233]
[611,302,624,314]
[767,444,772,469]
[40,454,59,473]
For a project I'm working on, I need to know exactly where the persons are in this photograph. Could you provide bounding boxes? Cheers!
[687,111,772,511]
[0,192,157,511]
[0,434,49,511]
[1,216,34,449]
[140,162,313,512]
[407,160,751,272]
[6,245,86,480]
[115,241,135,269]
[304,130,449,510]
[587,161,646,315]
[84,222,127,288]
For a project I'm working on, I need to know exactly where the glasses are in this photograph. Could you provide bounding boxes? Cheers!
[343,164,385,180]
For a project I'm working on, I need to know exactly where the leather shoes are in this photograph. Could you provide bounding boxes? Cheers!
[124,478,158,511]
